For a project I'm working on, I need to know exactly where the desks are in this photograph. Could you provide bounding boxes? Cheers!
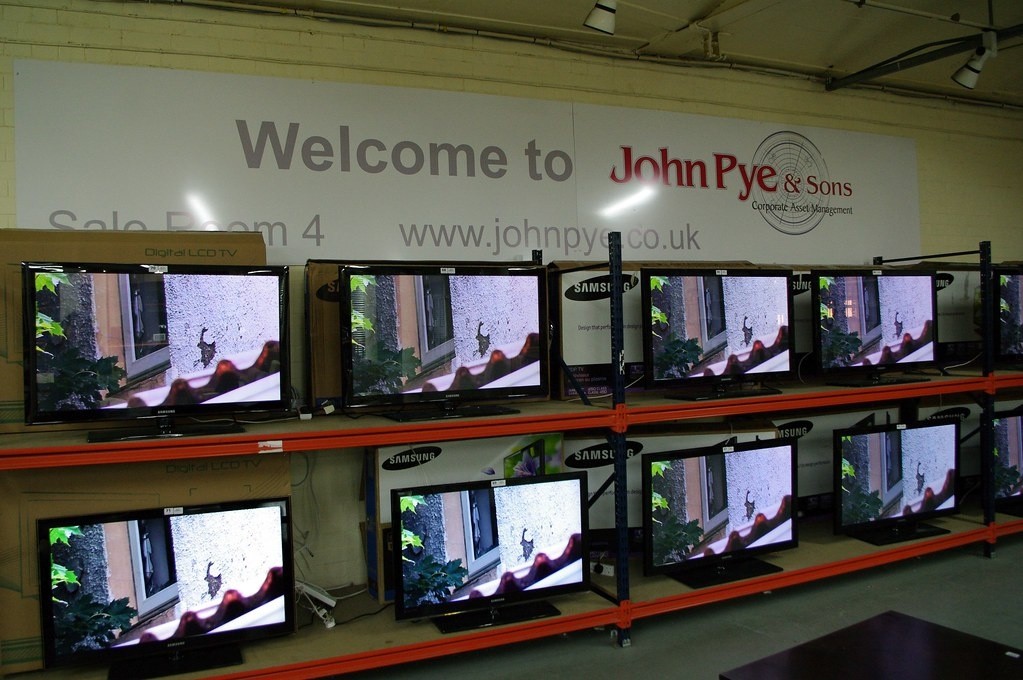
[716,610,1023,680]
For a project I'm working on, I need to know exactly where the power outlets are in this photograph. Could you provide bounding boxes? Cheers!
[589,542,608,558]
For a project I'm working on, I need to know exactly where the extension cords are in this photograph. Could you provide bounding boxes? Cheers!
[293,581,337,608]
[296,405,313,421]
[590,561,615,576]
[312,405,335,416]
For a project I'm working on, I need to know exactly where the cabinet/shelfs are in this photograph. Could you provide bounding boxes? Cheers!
[0,242,1023,680]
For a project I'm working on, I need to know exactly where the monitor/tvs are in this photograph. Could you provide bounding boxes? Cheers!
[979,408,1023,518]
[642,436,800,590]
[389,470,590,635]
[992,267,1023,371]
[811,269,941,387]
[833,417,962,546]
[37,496,295,680]
[20,260,291,441]
[337,264,550,422]
[639,268,797,401]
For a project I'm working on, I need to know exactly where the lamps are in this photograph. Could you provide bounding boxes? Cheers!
[583,0,618,35]
[951,45,991,90]
